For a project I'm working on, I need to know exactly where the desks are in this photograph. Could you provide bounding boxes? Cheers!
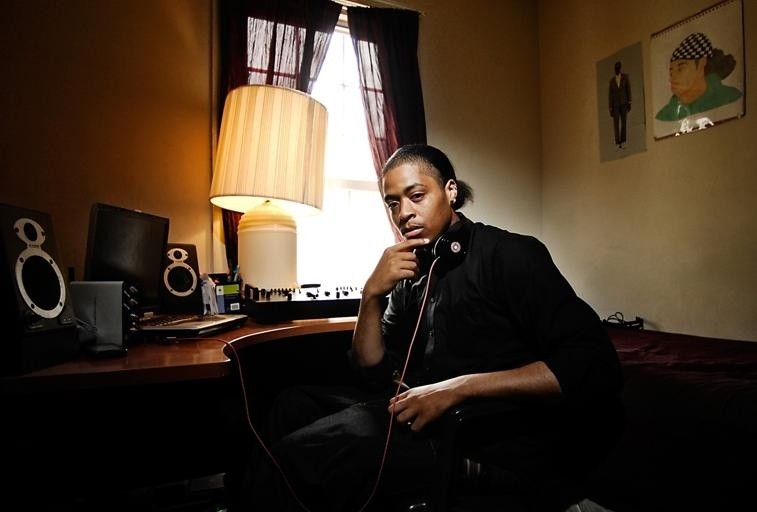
[0,296,754,511]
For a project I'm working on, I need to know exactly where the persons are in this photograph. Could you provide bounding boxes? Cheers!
[655,31,741,124]
[606,60,632,149]
[276,145,622,507]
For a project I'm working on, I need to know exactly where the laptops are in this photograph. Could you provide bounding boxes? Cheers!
[83,202,248,338]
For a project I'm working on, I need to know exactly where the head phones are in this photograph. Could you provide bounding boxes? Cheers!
[413,219,474,272]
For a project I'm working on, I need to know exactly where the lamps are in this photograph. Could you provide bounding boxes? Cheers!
[207,82,330,295]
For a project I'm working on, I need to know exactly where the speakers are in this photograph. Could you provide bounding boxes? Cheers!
[163,243,205,316]
[1,207,76,336]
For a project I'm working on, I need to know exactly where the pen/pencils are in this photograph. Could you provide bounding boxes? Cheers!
[234,265,240,281]
